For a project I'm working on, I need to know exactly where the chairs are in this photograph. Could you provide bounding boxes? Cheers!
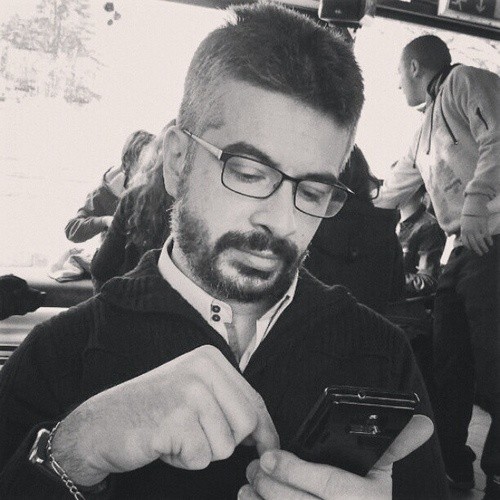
[304,208,400,316]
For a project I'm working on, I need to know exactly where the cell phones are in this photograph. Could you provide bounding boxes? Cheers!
[287,387,421,476]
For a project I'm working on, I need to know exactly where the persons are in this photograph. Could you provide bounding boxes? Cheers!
[87,119,180,295]
[0,2,452,500]
[65,130,161,299]
[387,161,446,322]
[369,32,499,499]
[302,144,406,322]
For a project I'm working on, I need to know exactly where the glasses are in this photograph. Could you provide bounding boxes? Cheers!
[183,128,356,220]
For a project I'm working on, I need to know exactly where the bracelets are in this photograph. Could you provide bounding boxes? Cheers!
[30,416,112,500]
